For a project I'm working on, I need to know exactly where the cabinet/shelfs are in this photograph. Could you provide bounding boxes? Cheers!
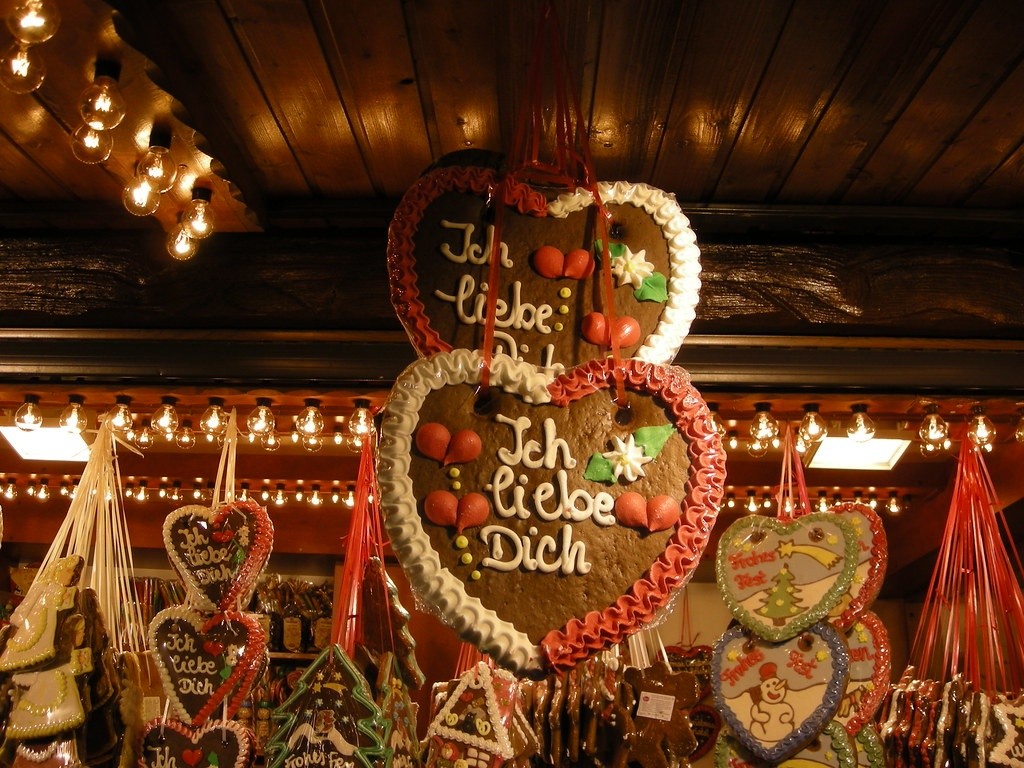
[221,637,327,768]
